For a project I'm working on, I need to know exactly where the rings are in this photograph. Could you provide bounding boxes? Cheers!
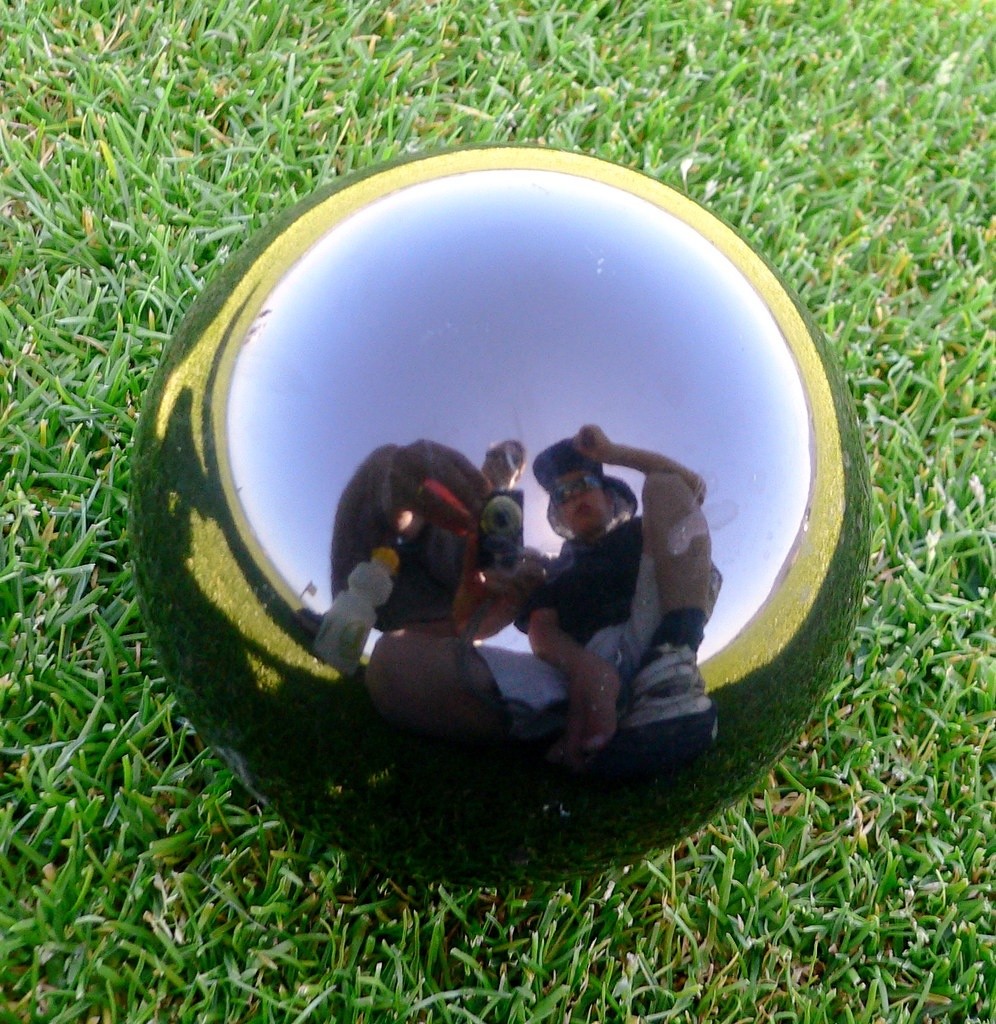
[413,483,425,500]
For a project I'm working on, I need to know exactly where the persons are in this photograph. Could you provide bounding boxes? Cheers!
[295,435,546,675]
[362,424,725,779]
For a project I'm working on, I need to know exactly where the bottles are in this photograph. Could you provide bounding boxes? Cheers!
[312,547,398,674]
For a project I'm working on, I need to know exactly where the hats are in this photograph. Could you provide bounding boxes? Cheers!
[534,437,603,497]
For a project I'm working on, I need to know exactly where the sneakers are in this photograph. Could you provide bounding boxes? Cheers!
[586,633,718,775]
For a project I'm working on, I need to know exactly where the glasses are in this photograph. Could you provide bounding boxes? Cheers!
[486,449,518,467]
[549,472,602,504]
